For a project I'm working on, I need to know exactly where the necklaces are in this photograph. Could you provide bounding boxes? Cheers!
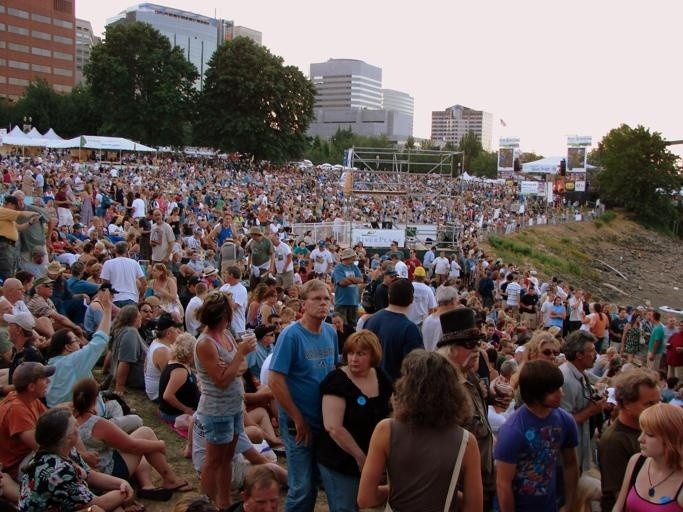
[645,459,681,496]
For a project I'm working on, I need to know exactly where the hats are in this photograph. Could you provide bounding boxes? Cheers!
[414,267,426,277]
[341,248,356,258]
[12,362,56,385]
[248,227,263,236]
[45,261,66,275]
[317,240,325,247]
[437,287,458,302]
[34,276,55,285]
[436,308,486,346]
[154,312,175,331]
[4,312,33,331]
[4,195,19,209]
[203,266,218,277]
[385,269,398,275]
[98,282,120,294]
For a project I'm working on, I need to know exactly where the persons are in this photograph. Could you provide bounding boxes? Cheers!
[147,263,184,321]
[185,283,208,338]
[0,470,20,504]
[100,242,146,308]
[148,143,331,284]
[666,321,683,379]
[194,290,258,509]
[657,316,679,370]
[156,333,202,425]
[666,388,683,406]
[495,360,580,512]
[317,330,394,512]
[42,262,90,323]
[489,362,519,404]
[144,294,161,317]
[407,267,438,330]
[245,269,333,385]
[136,303,156,343]
[432,308,494,512]
[360,271,383,314]
[371,270,399,311]
[458,269,588,361]
[243,369,279,425]
[179,279,200,310]
[422,286,459,354]
[0,362,64,471]
[596,365,664,511]
[144,314,182,403]
[99,152,149,242]
[509,331,567,408]
[661,377,679,402]
[588,292,646,379]
[364,277,424,384]
[101,304,148,397]
[180,407,290,491]
[0,148,100,280]
[67,263,99,299]
[19,407,148,512]
[612,404,683,512]
[46,289,144,435]
[0,296,54,367]
[3,277,55,340]
[332,248,364,330]
[14,270,35,299]
[357,349,484,512]
[330,313,357,364]
[226,464,280,512]
[26,274,87,344]
[200,268,223,293]
[2,314,48,405]
[83,283,118,341]
[646,312,665,371]
[268,283,340,512]
[240,398,289,457]
[487,382,515,434]
[557,331,613,476]
[67,378,195,500]
[444,276,456,287]
[464,173,600,268]
[331,169,464,268]
[175,492,220,512]
[220,266,247,345]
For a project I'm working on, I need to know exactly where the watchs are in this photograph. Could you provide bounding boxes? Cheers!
[87,506,92,512]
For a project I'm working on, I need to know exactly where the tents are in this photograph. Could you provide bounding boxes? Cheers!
[52,135,156,153]
[520,156,598,174]
[2,126,64,157]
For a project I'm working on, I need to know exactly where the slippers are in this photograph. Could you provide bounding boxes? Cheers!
[172,479,192,492]
[136,488,171,501]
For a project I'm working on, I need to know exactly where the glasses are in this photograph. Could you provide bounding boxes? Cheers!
[71,336,80,344]
[140,309,154,314]
[537,348,560,357]
[274,321,283,326]
[453,341,482,349]
[45,284,53,288]
[265,333,274,337]
